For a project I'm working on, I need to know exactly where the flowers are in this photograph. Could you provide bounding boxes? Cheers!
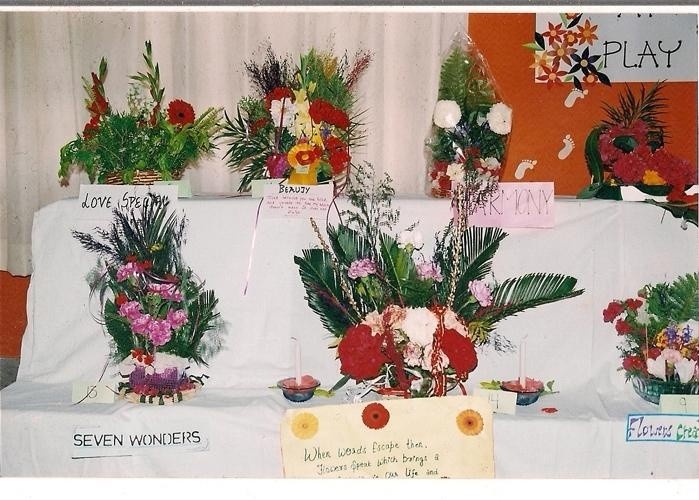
[212,30,375,192]
[58,40,227,188]
[427,46,513,200]
[70,191,228,406]
[577,79,699,208]
[603,272,699,385]
[294,163,583,396]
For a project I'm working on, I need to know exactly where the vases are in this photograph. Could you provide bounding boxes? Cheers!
[101,169,166,185]
[250,173,347,201]
[628,369,699,406]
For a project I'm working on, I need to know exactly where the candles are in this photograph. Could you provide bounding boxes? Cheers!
[295,335,302,386]
[520,340,526,390]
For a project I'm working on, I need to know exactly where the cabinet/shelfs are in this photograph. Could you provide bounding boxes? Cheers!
[1,196,699,478]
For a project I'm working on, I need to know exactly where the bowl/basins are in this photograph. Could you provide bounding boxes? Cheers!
[277,378,320,404]
[501,380,544,407]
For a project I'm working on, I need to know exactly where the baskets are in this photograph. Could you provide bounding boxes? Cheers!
[96,167,182,185]
